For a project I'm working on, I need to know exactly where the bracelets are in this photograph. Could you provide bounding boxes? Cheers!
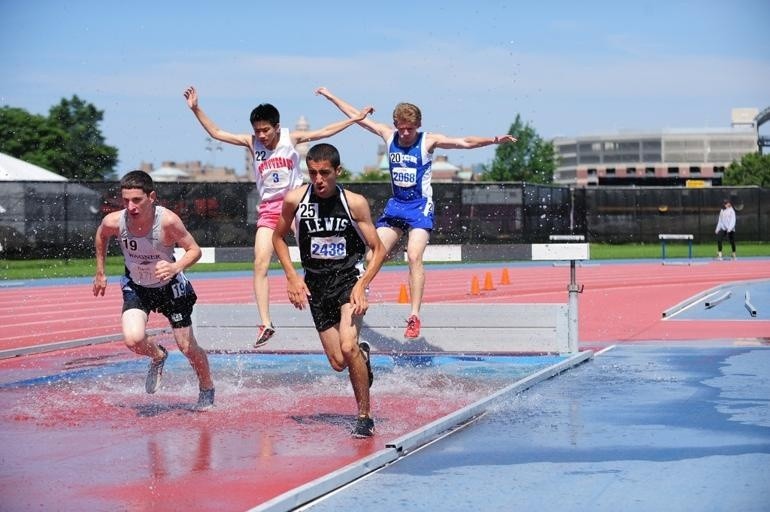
[494,136,499,144]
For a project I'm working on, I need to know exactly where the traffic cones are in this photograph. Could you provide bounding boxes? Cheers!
[499,269,511,284]
[398,284,409,304]
[470,276,484,296]
[484,272,496,290]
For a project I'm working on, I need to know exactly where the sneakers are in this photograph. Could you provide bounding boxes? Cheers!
[254,321,276,349]
[351,414,376,439]
[195,387,215,413]
[145,344,168,395]
[358,341,374,389]
[403,315,422,339]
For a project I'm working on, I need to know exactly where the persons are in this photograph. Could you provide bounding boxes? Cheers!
[90,169,216,412]
[307,86,517,340]
[182,84,375,348]
[714,198,737,260]
[270,144,389,440]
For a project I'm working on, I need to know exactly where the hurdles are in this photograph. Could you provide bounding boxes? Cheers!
[548,235,585,266]
[169,242,591,355]
[659,233,695,266]
